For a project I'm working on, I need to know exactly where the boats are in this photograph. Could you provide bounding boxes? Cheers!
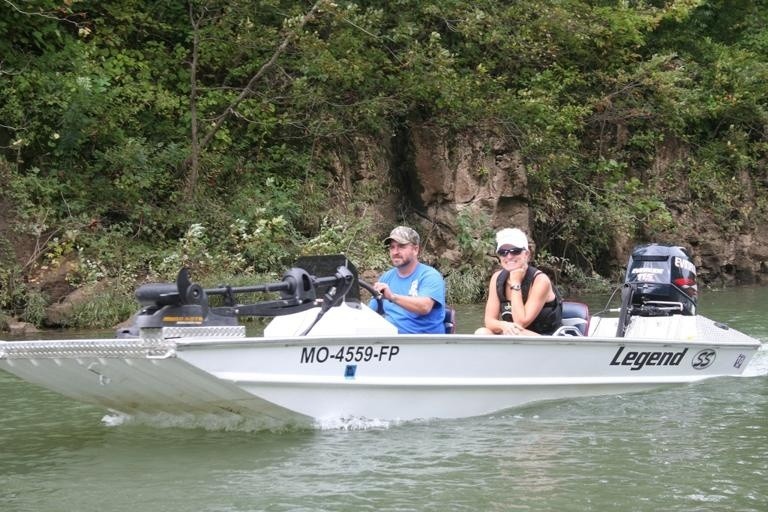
[0,242,763,431]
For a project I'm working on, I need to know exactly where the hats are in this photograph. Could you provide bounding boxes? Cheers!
[496,227,528,253]
[384,226,420,245]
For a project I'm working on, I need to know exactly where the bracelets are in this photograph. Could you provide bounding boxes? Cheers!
[390,294,397,303]
[511,284,521,290]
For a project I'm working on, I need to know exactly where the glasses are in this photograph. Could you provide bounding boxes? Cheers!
[498,248,522,256]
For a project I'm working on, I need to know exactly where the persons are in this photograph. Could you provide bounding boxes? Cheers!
[367,224,446,336]
[475,227,562,336]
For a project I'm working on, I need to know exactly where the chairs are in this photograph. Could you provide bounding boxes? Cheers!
[440,300,591,336]
